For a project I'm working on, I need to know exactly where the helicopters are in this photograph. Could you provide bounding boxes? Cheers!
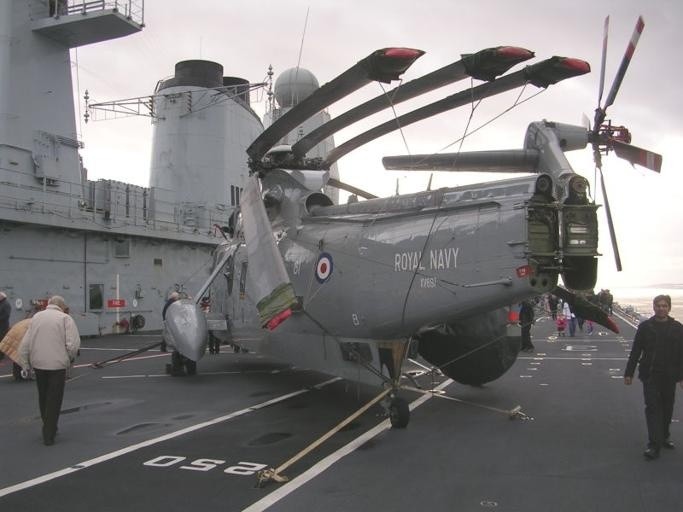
[164,15,666,426]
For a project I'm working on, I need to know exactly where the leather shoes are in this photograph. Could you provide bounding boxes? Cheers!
[663,438,674,448]
[643,447,658,457]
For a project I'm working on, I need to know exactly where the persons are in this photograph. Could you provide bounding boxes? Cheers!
[517,286,613,353]
[623,293,683,460]
[17,296,82,446]
[0,292,13,360]
[159,290,249,356]
